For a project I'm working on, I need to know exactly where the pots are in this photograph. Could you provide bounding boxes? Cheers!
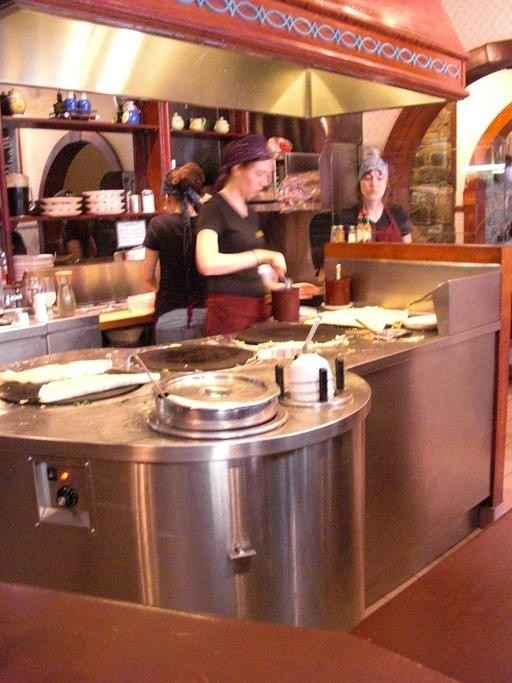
[145,371,281,429]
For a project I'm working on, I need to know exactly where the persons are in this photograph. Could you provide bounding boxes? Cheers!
[46,184,99,264]
[136,159,211,346]
[339,143,416,245]
[195,131,290,341]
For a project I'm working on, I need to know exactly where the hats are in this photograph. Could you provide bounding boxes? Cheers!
[357,159,388,183]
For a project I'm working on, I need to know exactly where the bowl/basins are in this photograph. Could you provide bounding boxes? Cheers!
[288,353,334,403]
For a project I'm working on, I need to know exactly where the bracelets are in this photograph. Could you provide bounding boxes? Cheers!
[252,249,262,265]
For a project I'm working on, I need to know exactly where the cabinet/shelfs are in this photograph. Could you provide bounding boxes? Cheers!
[1,314,102,365]
[0,100,312,283]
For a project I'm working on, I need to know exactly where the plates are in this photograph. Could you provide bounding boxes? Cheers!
[320,302,356,310]
[12,253,52,283]
[402,314,439,332]
[40,187,124,217]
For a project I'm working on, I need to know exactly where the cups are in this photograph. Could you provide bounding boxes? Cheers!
[6,172,35,220]
[189,117,206,131]
[270,288,299,322]
[323,277,349,304]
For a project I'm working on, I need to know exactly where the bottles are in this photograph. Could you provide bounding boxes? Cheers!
[54,270,77,318]
[170,112,184,129]
[127,189,154,213]
[64,91,90,113]
[121,99,140,123]
[330,211,372,245]
[214,115,229,133]
[7,89,25,118]
